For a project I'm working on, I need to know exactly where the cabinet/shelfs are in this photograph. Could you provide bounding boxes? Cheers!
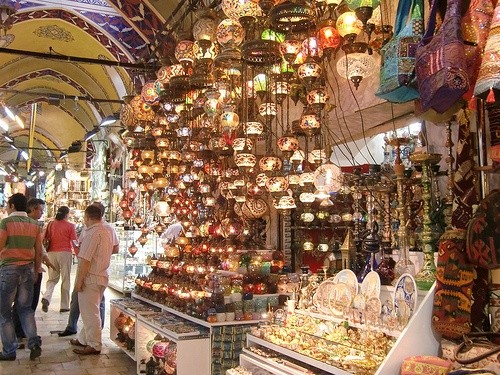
[107,229,168,296]
[136,312,210,375]
[224,283,440,375]
[110,298,156,361]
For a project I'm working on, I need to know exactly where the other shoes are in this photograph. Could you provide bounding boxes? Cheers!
[60,309,70,312]
[0,351,17,361]
[57,327,77,336]
[41,299,49,313]
[72,345,101,355]
[30,344,42,359]
[70,339,87,346]
[16,337,26,349]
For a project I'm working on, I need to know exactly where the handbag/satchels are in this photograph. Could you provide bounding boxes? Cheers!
[412,39,483,125]
[440,330,500,375]
[42,221,52,251]
[412,1,470,115]
[374,0,425,104]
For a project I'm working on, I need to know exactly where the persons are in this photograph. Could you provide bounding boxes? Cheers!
[0,193,56,360]
[56,202,119,354]
[41,206,79,313]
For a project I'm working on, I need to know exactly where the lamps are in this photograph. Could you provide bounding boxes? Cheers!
[0,0,392,365]
